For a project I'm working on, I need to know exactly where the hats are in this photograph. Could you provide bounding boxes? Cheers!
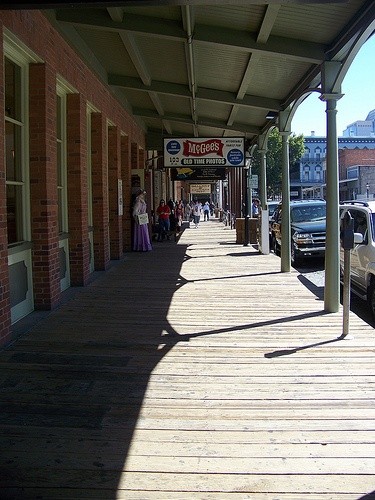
[135,189,144,197]
[205,201,209,205]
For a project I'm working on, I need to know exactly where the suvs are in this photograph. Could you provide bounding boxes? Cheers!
[339,200,375,315]
[267,199,325,268]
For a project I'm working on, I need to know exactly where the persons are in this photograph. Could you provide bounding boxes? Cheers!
[156,199,171,242]
[175,200,216,229]
[132,188,152,251]
[252,200,259,218]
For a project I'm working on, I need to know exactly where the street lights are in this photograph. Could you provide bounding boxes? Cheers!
[243,147,253,247]
[366,183,370,198]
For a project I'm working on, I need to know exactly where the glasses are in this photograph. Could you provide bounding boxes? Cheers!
[160,202,164,203]
[142,193,146,195]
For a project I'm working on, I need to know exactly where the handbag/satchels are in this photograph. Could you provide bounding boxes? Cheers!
[138,213,149,225]
